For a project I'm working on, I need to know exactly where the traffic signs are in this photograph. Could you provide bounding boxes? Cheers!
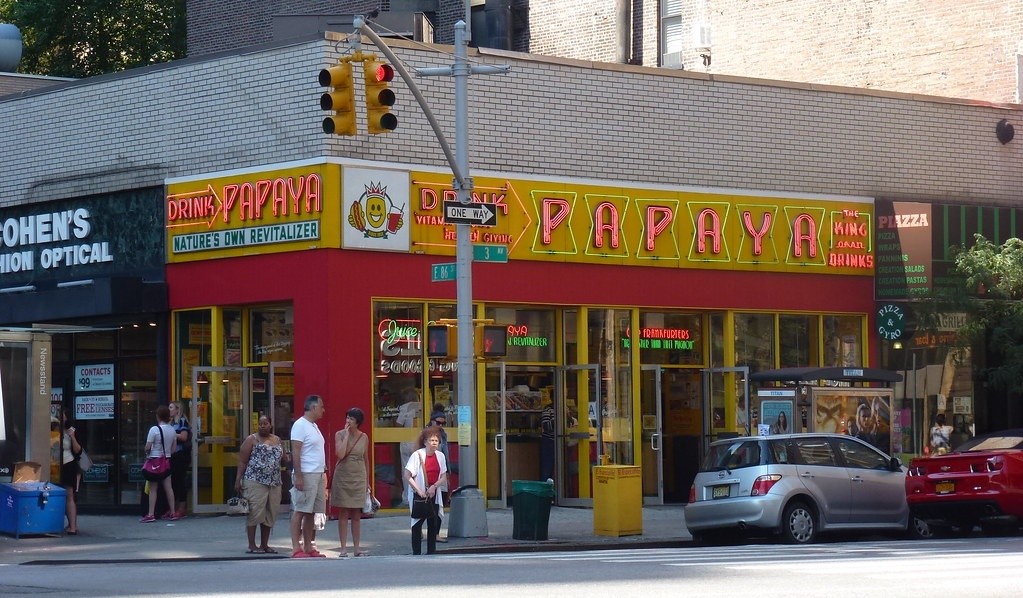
[443,200,498,226]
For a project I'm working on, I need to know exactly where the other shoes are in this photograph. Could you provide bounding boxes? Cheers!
[340,553,347,556]
[394,503,409,509]
[354,553,365,557]
[63,528,77,535]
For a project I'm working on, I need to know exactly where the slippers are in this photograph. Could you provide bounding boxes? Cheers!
[290,551,311,558]
[246,548,265,554]
[309,550,326,557]
[265,548,278,553]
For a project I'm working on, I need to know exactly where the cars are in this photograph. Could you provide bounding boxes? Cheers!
[904,430,1023,534]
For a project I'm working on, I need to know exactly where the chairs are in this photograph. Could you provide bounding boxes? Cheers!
[728,454,741,468]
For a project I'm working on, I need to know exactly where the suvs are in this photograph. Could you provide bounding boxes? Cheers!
[685,433,910,544]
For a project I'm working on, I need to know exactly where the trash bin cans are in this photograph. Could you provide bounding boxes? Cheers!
[511,480,554,540]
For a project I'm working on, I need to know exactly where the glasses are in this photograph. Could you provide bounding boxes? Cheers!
[434,419,447,426]
[860,415,871,417]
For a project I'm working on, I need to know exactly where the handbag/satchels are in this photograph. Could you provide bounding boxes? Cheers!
[74,447,93,472]
[142,457,172,482]
[326,467,334,489]
[412,497,437,518]
[227,490,250,516]
[362,489,372,514]
[182,432,205,450]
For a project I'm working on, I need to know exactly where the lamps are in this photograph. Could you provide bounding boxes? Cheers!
[893,338,902,350]
[196,312,209,384]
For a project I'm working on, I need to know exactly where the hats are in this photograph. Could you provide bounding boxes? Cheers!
[51,416,60,422]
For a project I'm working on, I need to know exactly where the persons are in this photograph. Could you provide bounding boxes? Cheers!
[736,394,751,428]
[288,395,328,558]
[770,395,889,463]
[404,404,451,555]
[235,415,292,553]
[53,400,193,535]
[330,408,373,557]
[930,414,954,454]
[540,388,574,506]
[395,387,424,510]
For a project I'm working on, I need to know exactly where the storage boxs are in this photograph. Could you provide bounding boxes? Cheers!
[11,460,41,483]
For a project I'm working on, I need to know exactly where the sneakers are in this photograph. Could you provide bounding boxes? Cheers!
[176,511,189,519]
[161,510,175,521]
[138,515,156,523]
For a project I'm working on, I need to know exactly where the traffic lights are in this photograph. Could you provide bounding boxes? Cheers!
[318,63,356,135]
[474,323,508,357]
[364,61,397,135]
[427,324,456,359]
